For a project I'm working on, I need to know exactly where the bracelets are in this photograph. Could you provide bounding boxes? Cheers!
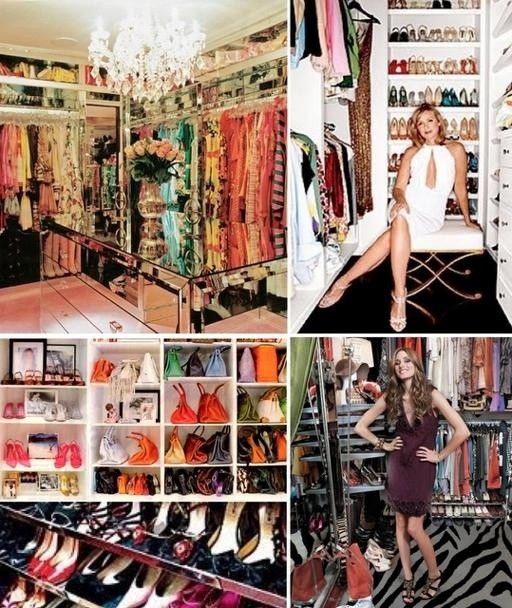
[377,438,385,450]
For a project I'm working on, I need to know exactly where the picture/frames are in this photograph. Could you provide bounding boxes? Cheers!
[118,389,161,424]
[8,337,48,384]
[23,388,59,417]
[45,343,77,381]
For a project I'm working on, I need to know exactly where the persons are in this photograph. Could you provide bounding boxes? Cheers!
[105,403,118,418]
[318,101,480,333]
[354,347,471,604]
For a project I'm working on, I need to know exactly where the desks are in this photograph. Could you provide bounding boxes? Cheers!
[33,204,286,330]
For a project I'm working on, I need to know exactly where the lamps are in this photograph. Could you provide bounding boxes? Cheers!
[83,0,210,109]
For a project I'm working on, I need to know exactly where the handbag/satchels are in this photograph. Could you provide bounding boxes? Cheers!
[163,345,228,376]
[95,469,160,495]
[238,346,286,382]
[238,468,286,492]
[139,352,160,384]
[164,468,234,494]
[162,426,186,465]
[88,351,114,384]
[292,543,330,601]
[170,383,197,423]
[128,429,158,465]
[187,426,206,466]
[198,383,226,423]
[237,430,286,462]
[237,386,287,423]
[97,426,128,465]
[335,542,373,599]
[208,427,230,463]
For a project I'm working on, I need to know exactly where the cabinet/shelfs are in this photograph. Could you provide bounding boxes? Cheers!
[0,501,288,607]
[0,331,288,506]
[485,0,512,262]
[386,2,486,225]
[490,131,512,329]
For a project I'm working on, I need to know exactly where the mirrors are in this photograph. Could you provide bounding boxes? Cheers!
[291,337,342,607]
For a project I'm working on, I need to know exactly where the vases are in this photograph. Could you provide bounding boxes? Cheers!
[138,222,168,264]
[137,176,168,221]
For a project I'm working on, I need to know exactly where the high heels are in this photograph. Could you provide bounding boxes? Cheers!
[387,84,479,216]
[44,403,68,422]
[345,461,383,486]
[402,575,415,603]
[4,401,26,419]
[352,516,397,573]
[384,484,505,518]
[320,280,353,310]
[0,368,85,385]
[55,441,83,497]
[389,54,479,75]
[386,1,479,10]
[5,439,33,468]
[0,502,285,608]
[388,23,478,43]
[420,570,442,599]
[390,287,408,333]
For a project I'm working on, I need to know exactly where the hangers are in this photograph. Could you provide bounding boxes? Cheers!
[345,0,381,26]
[201,83,286,121]
[323,122,356,154]
[460,419,503,436]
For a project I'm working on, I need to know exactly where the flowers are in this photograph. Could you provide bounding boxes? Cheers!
[119,138,188,186]
